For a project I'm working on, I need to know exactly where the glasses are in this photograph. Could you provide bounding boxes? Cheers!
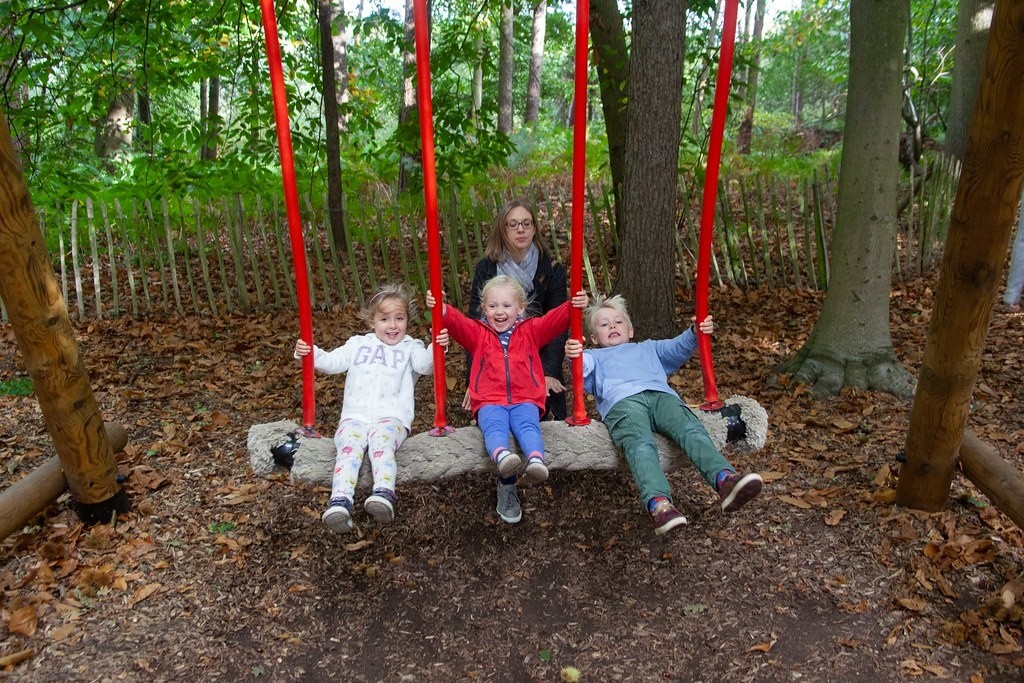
[506,219,533,230]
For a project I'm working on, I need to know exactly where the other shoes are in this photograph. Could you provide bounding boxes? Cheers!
[526,456,549,481]
[719,472,763,513]
[364,487,398,522]
[496,450,521,474]
[652,500,688,536]
[322,496,353,533]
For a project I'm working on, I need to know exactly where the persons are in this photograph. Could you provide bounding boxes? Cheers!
[292,287,449,535]
[462,201,566,522]
[564,291,764,536]
[425,291,589,488]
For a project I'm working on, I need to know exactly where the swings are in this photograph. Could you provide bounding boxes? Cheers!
[249,0,767,483]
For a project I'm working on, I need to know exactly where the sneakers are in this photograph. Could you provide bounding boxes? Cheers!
[495,482,522,523]
